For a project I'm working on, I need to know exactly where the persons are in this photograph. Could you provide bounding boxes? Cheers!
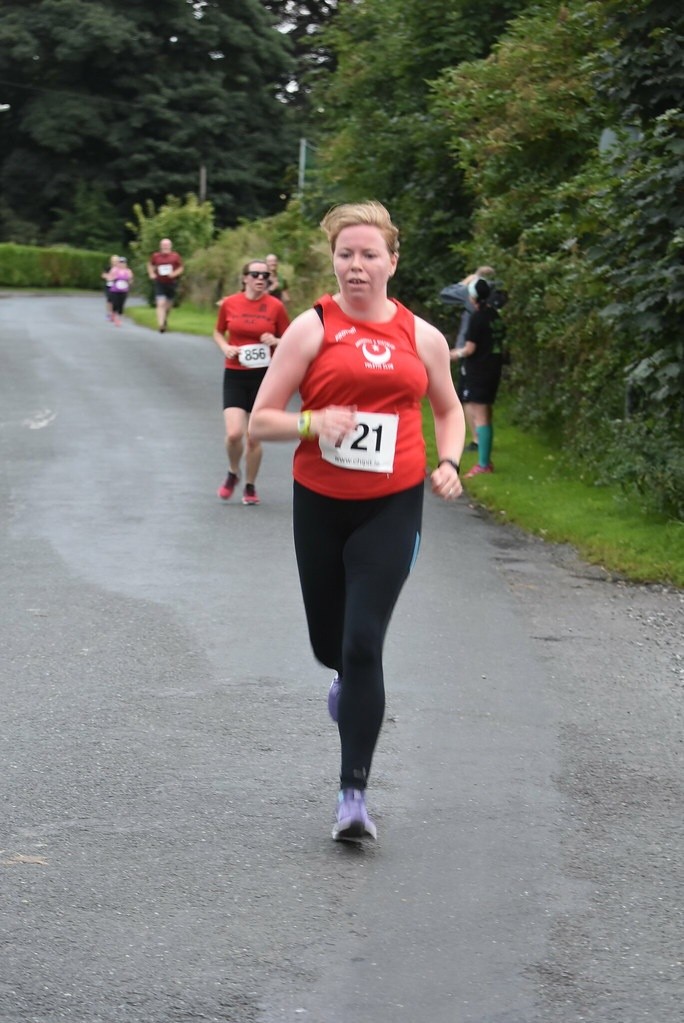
[214,252,289,503]
[145,237,185,331]
[246,195,465,841]
[450,277,503,478]
[100,253,132,326]
[440,265,508,452]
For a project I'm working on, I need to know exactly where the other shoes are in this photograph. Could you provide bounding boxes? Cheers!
[466,442,478,451]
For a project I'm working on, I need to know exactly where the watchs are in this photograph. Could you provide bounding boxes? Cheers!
[297,409,311,442]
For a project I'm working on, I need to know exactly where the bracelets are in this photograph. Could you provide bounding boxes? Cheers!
[435,458,461,475]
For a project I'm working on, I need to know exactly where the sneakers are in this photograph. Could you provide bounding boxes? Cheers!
[243,483,260,504]
[462,459,494,477]
[331,787,377,843]
[219,469,241,499]
[328,674,341,724]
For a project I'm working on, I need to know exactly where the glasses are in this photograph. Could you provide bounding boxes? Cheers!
[245,271,271,279]
